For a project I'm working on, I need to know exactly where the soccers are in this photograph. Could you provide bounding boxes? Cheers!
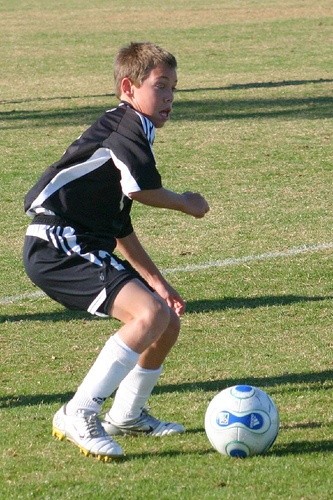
[205,384,278,456]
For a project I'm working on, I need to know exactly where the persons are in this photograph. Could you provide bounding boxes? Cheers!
[21,39,213,461]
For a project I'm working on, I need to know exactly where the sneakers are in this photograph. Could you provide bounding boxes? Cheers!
[52,403,126,462]
[102,407,185,436]
